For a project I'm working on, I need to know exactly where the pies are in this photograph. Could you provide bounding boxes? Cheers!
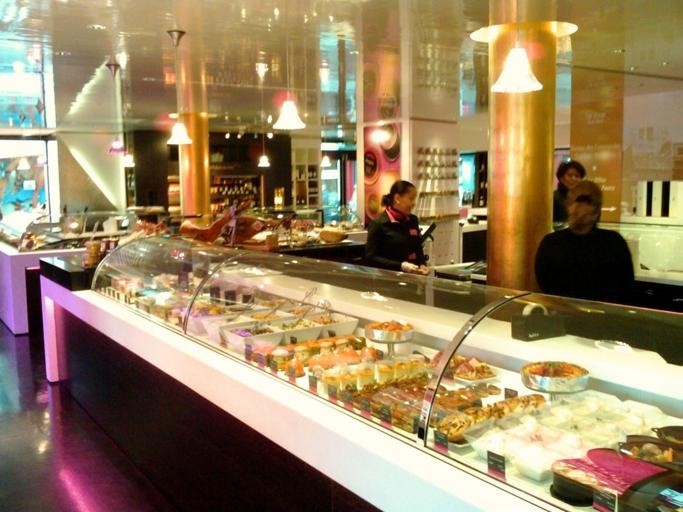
[438,395,547,442]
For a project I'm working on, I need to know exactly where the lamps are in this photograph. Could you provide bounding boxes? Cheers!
[487,3,546,95]
[163,28,193,146]
[270,1,309,131]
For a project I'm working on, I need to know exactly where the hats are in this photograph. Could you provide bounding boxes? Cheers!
[564,181,603,205]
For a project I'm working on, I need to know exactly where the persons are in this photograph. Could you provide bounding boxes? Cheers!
[366,180,431,303]
[533,181,634,305]
[553,160,586,222]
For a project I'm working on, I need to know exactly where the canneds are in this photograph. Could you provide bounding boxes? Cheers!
[266,235,279,253]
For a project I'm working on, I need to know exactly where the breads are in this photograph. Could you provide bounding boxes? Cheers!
[307,346,379,370]
